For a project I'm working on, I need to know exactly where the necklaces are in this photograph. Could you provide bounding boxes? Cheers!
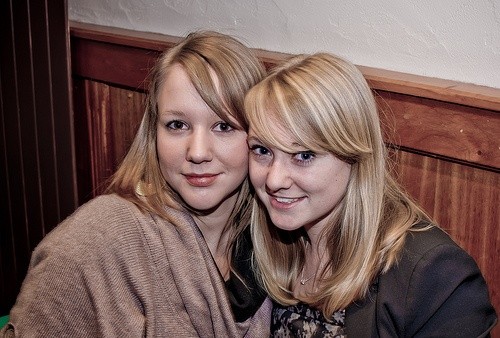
[300,240,315,285]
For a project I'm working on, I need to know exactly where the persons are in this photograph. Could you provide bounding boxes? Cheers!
[1,29,275,338]
[242,51,498,337]
